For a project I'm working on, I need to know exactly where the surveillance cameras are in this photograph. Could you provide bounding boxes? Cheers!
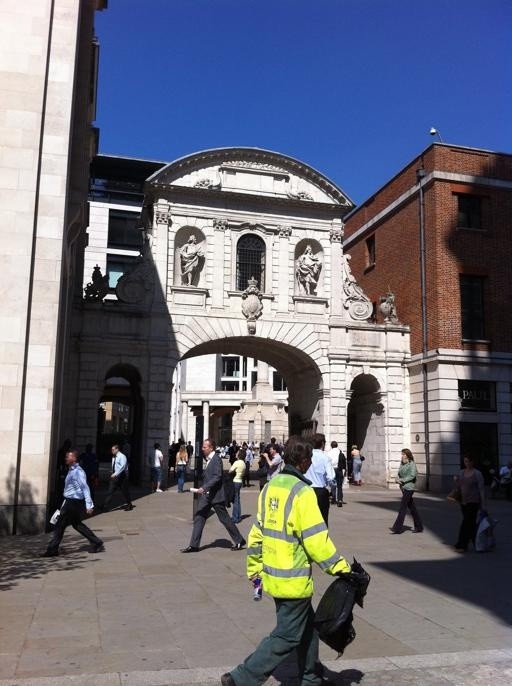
[430,129,436,135]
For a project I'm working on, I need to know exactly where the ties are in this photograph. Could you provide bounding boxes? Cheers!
[112,457,116,473]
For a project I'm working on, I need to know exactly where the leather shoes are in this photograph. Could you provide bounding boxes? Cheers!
[180,546,199,552]
[87,541,103,553]
[39,551,58,558]
[232,539,246,551]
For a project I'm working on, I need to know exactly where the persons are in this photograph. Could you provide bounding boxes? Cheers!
[169,433,346,552]
[388,449,424,534]
[297,245,321,294]
[448,452,512,553]
[180,235,203,285]
[58,438,72,499]
[77,443,99,509]
[40,448,103,557]
[150,443,164,493]
[99,444,137,511]
[220,435,352,686]
[103,434,131,459]
[350,444,362,486]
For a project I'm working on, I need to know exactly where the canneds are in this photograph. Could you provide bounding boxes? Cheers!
[253,578,262,601]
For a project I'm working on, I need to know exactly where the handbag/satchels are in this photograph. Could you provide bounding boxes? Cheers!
[446,485,462,505]
